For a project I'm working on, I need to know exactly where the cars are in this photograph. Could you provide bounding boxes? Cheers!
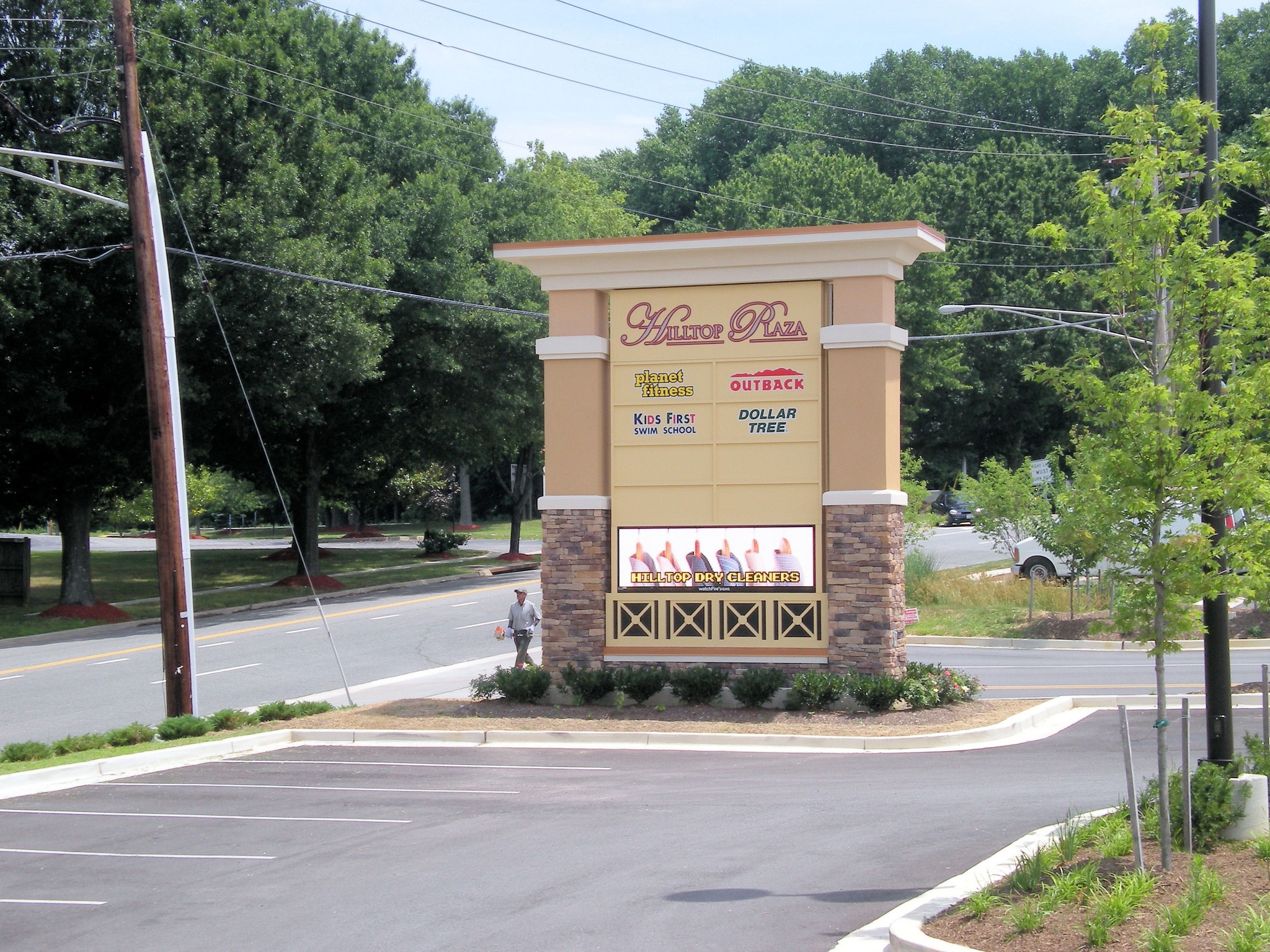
[1028,480,1075,514]
[930,491,990,527]
[1009,487,1249,586]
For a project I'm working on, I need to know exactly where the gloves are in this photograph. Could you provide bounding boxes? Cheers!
[506,628,513,638]
[527,624,534,633]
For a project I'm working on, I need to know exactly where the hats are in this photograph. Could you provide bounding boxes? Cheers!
[514,587,527,595]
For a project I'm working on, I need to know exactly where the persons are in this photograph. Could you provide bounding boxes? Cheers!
[507,587,542,668]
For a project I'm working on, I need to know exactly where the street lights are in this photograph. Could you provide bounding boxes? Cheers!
[938,301,1176,448]
